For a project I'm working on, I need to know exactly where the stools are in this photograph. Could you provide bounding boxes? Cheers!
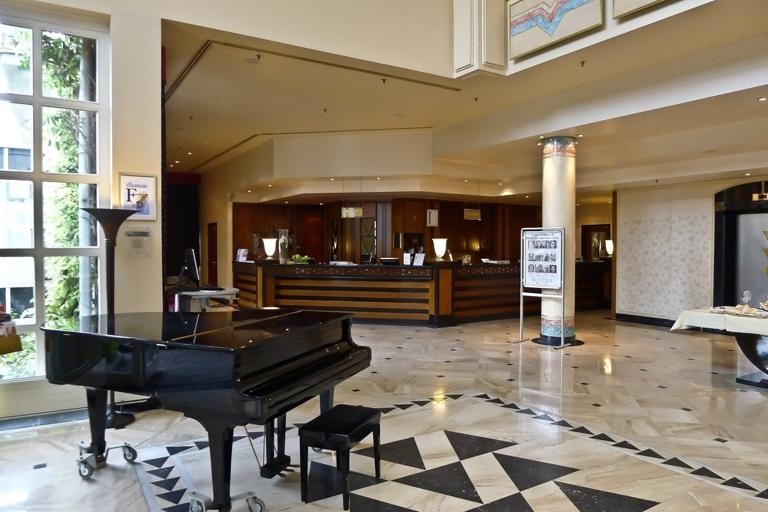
[297,403,381,509]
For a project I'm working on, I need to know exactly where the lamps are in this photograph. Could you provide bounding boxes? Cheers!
[605,240,613,256]
[262,238,278,259]
[464,179,482,222]
[432,238,447,259]
[341,175,363,218]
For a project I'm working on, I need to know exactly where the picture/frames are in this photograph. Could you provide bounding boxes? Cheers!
[118,171,157,221]
[508,0,667,62]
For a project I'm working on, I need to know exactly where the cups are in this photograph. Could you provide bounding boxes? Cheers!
[740,288,752,304]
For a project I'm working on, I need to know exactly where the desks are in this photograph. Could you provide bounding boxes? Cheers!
[670,306,768,387]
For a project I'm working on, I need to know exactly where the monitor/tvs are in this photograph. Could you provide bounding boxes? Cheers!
[174,249,200,291]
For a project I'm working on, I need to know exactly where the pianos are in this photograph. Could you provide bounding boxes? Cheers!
[39,309,371,512]
